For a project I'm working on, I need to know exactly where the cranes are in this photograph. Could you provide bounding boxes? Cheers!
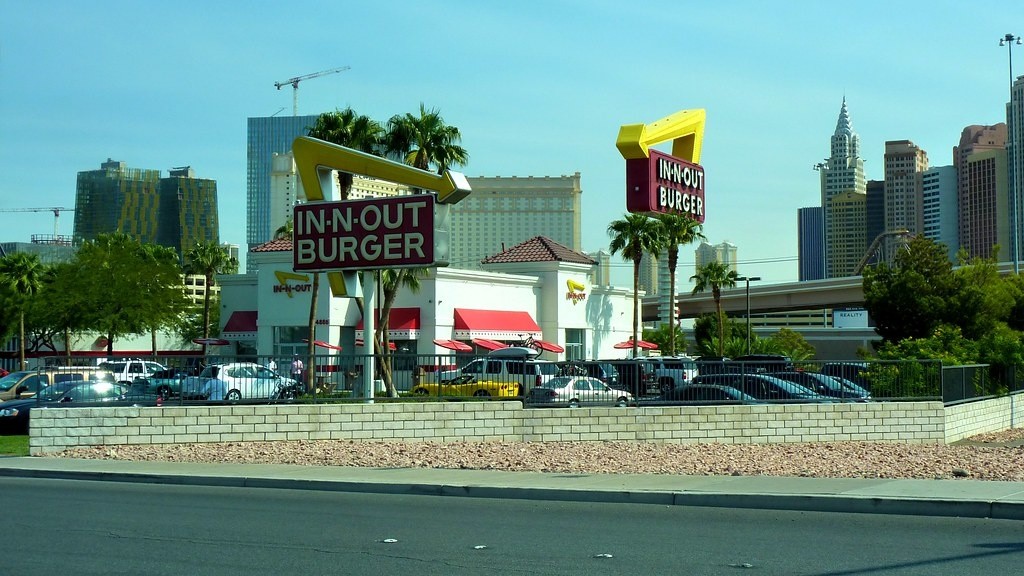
[0,207,76,241]
[275,65,352,115]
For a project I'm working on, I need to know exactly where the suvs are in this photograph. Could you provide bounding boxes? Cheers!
[633,356,699,394]
[437,346,560,397]
[599,360,659,395]
[99,361,168,388]
[555,360,621,389]
[722,355,795,371]
[0,367,120,403]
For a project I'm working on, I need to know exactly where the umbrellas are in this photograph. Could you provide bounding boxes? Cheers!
[531,339,564,353]
[193,338,230,345]
[302,339,342,351]
[614,340,658,349]
[474,338,508,350]
[433,340,473,352]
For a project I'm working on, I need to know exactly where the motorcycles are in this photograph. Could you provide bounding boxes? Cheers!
[268,373,306,404]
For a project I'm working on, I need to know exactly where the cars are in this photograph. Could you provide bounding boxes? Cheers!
[181,362,298,404]
[674,363,874,403]
[0,379,161,435]
[524,376,634,408]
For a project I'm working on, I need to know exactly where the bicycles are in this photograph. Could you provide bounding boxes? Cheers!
[509,333,543,359]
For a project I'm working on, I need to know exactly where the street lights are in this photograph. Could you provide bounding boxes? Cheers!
[813,161,830,328]
[999,32,1022,275]
[734,277,760,354]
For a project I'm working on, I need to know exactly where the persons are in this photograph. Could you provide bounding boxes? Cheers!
[201,367,227,399]
[265,357,277,371]
[290,354,303,379]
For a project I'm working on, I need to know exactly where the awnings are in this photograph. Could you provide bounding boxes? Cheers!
[221,311,258,339]
[355,307,420,340]
[454,308,543,340]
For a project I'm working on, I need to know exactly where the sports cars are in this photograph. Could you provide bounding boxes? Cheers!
[408,375,520,401]
[131,369,198,400]
